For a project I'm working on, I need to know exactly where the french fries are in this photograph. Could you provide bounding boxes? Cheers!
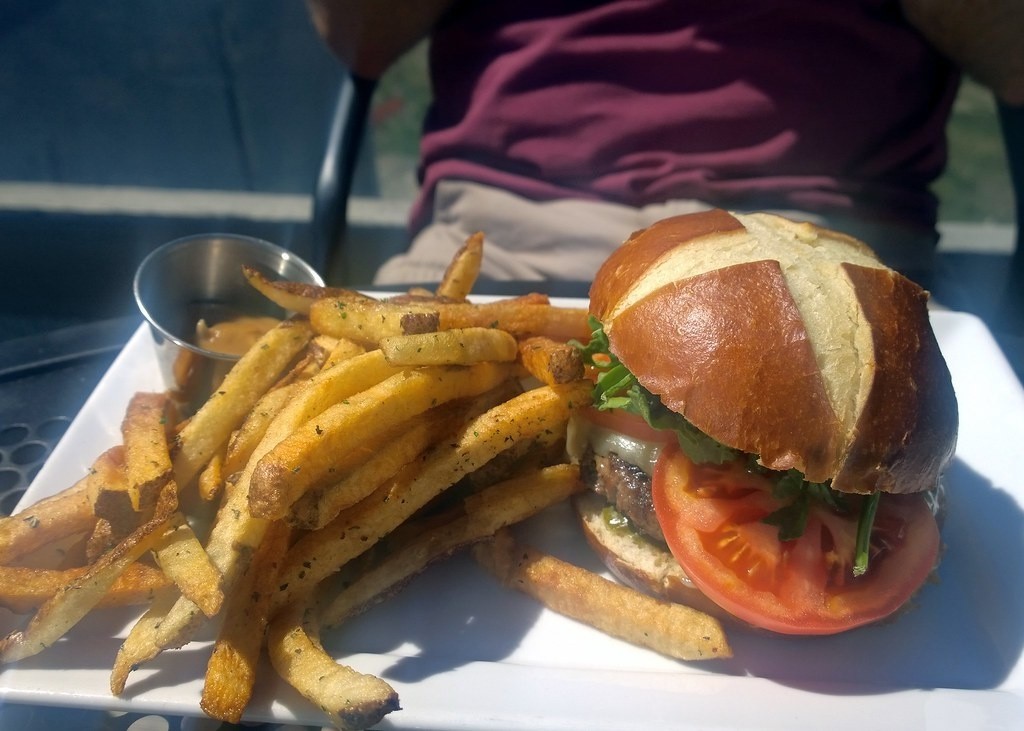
[0,231,734,731]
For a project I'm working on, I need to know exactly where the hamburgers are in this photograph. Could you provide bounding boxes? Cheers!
[566,209,958,636]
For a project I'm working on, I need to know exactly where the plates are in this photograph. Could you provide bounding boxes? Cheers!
[4,293,1024,728]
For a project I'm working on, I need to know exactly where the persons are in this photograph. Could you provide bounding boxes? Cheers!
[307,0,1024,291]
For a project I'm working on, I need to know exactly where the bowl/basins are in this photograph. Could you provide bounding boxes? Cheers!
[133,232,326,413]
